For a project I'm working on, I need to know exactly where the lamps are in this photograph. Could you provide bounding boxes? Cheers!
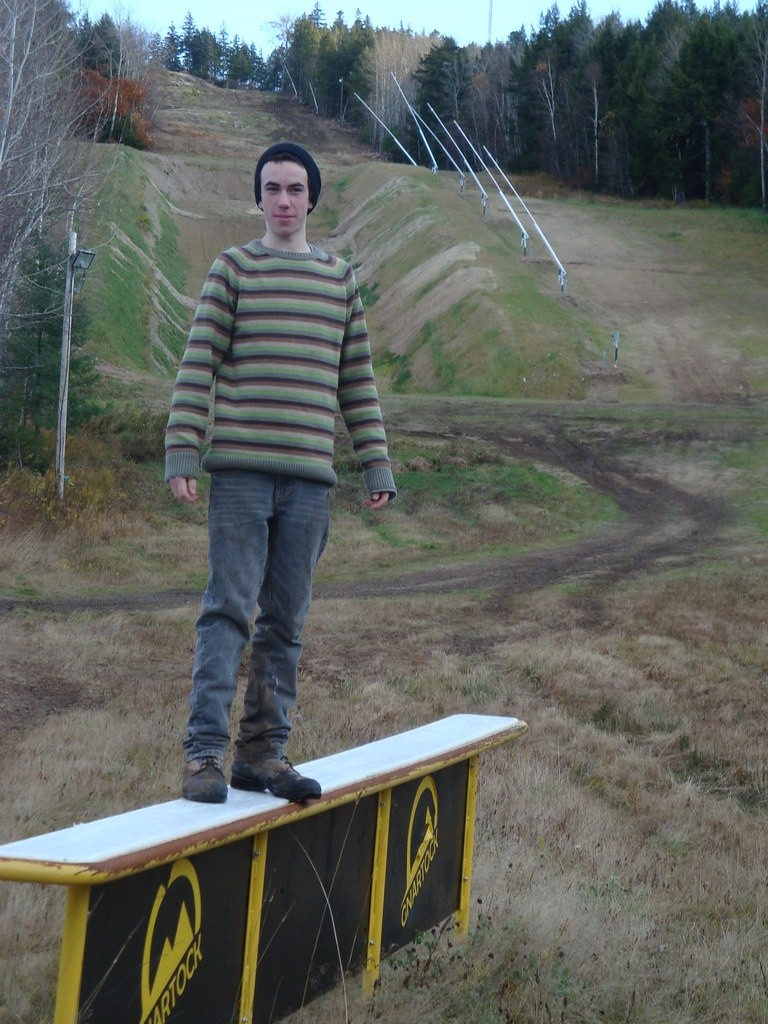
[73,277,86,295]
[70,249,96,270]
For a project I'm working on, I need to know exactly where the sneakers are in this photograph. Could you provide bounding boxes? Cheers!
[182,756,228,802]
[231,756,321,799]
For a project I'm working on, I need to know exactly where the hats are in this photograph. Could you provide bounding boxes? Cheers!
[255,143,321,215]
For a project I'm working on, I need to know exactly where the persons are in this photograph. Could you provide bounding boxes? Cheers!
[164,144,397,804]
